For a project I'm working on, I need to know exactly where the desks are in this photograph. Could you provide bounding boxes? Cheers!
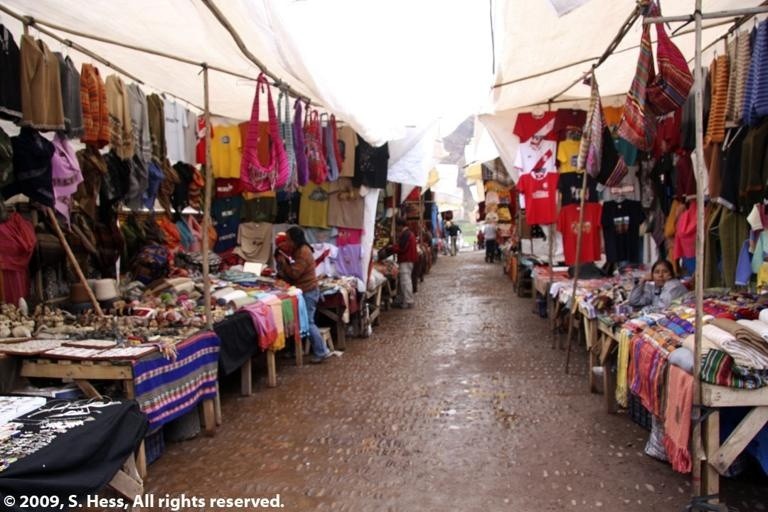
[510,262,768,512]
[0,266,401,511]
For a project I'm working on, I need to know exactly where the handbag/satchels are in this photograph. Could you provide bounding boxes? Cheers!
[239,118,344,192]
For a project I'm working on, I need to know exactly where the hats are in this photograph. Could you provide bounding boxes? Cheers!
[275,232,288,246]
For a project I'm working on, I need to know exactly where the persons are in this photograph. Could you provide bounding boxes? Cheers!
[476,218,503,263]
[275,227,329,364]
[628,259,690,306]
[387,218,416,309]
[444,219,462,257]
